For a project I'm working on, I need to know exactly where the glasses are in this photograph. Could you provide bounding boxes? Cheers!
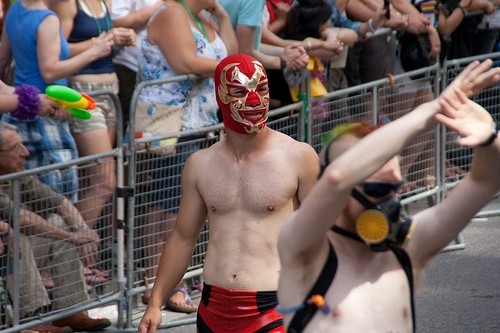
[359,180,408,199]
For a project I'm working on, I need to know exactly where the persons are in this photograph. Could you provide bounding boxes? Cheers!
[138,54,320,333]
[0,0,500,312]
[276,58,500,333]
[0,122,112,333]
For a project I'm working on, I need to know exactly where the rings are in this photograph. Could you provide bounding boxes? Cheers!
[435,48,437,50]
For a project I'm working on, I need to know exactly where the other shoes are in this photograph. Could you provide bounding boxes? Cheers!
[29,323,72,333]
[52,311,112,332]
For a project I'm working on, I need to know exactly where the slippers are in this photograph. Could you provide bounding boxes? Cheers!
[85,271,109,284]
[166,287,197,313]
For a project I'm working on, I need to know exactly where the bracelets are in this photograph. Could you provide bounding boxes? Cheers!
[459,6,465,15]
[368,18,376,33]
[404,15,409,27]
[279,56,286,70]
[480,116,499,147]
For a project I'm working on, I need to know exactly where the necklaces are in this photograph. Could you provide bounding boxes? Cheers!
[85,0,110,34]
[178,0,206,39]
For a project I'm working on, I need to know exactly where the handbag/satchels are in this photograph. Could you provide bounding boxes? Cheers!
[123,100,186,157]
